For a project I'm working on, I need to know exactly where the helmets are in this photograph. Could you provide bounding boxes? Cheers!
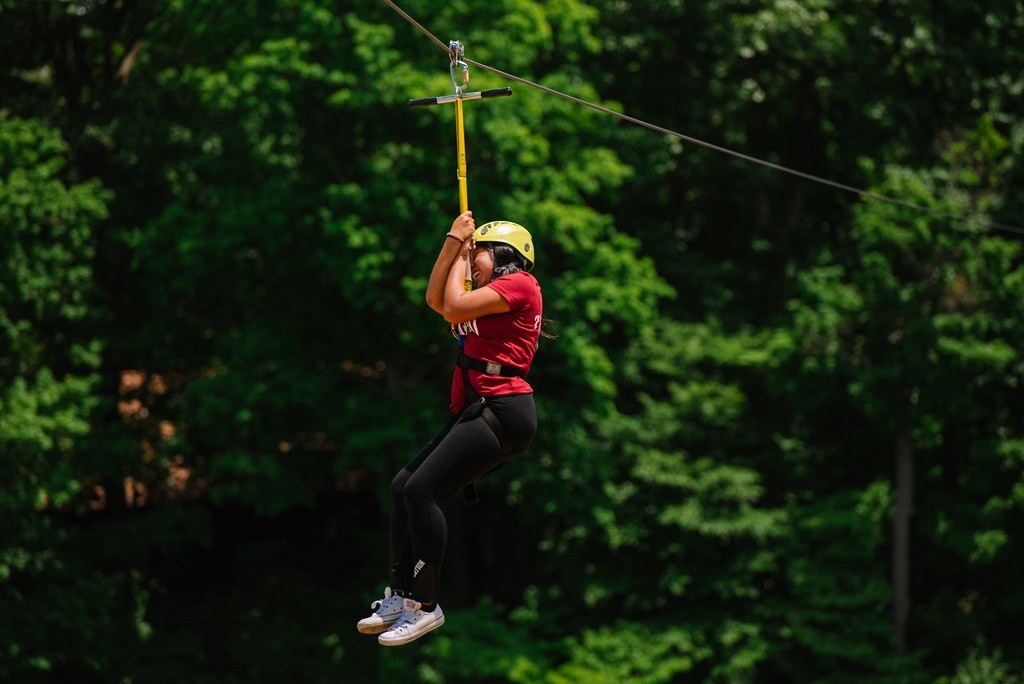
[473,221,535,271]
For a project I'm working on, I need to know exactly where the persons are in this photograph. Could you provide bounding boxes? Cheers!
[357,208,543,646]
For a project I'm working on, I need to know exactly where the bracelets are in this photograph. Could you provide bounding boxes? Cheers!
[447,232,464,243]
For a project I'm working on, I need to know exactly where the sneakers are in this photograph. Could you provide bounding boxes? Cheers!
[378,598,445,646]
[356,586,405,634]
